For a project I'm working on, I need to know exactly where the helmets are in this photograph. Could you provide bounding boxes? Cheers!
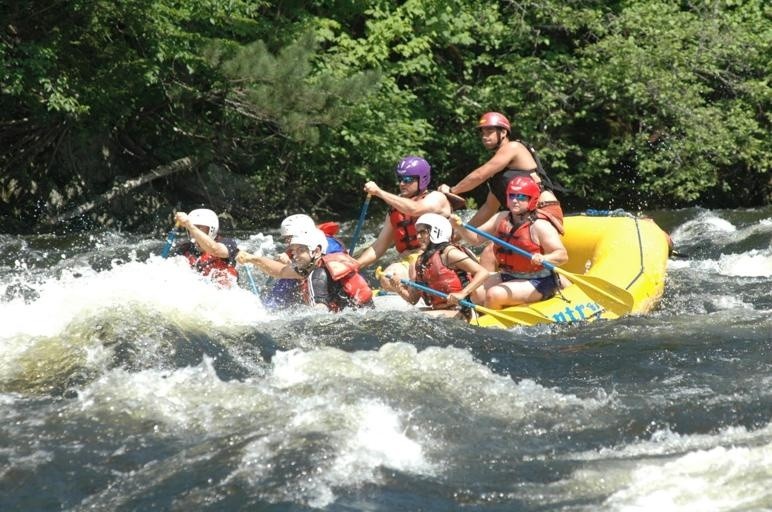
[291,227,328,255]
[275,214,314,240]
[186,208,220,245]
[476,112,511,136]
[506,175,541,211]
[414,213,453,244]
[395,157,431,190]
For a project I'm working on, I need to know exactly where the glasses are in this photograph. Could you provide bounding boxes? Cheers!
[509,194,527,201]
[398,175,415,183]
[417,228,431,238]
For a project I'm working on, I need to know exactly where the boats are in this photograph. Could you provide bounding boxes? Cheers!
[367,213,674,340]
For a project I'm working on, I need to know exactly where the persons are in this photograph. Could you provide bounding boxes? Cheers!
[391,213,488,322]
[356,156,453,281]
[265,213,348,306]
[438,112,555,243]
[452,176,569,317]
[235,229,376,313]
[173,207,237,286]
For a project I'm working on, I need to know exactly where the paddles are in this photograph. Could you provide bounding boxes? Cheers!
[454,217,633,319]
[374,267,554,330]
